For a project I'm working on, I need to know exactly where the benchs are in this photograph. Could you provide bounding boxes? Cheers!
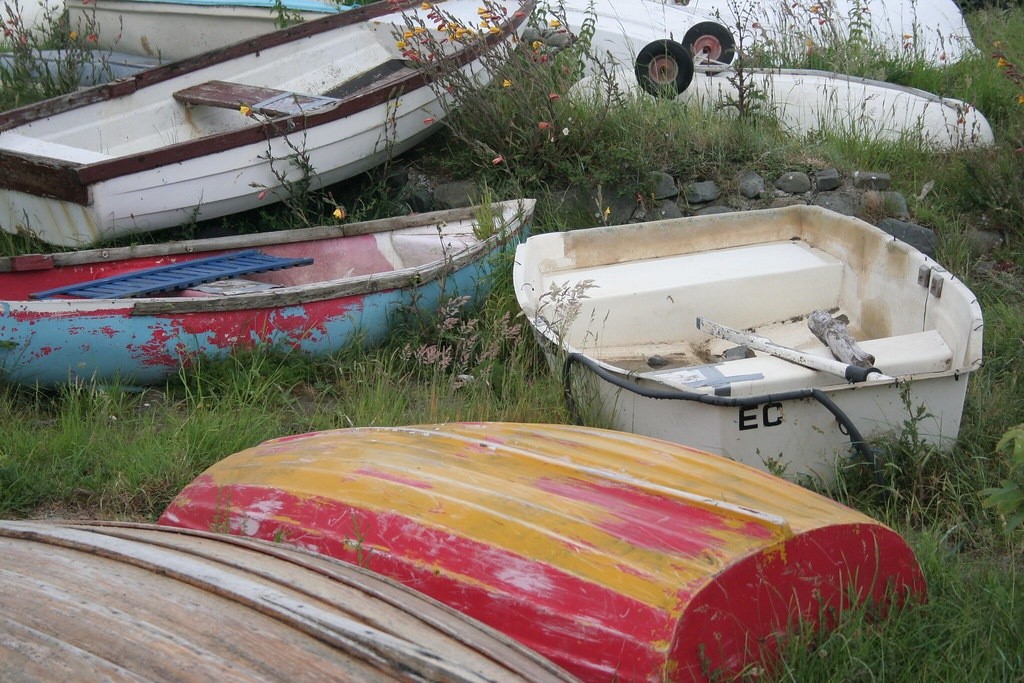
[155,278,285,298]
[172,80,343,119]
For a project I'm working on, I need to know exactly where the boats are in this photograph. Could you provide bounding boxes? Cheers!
[511,199,984,515]
[53,2,345,61]
[521,1,985,70]
[0,195,538,388]
[1,1,543,251]
[0,517,588,683]
[156,417,931,683]
[569,20,998,163]
